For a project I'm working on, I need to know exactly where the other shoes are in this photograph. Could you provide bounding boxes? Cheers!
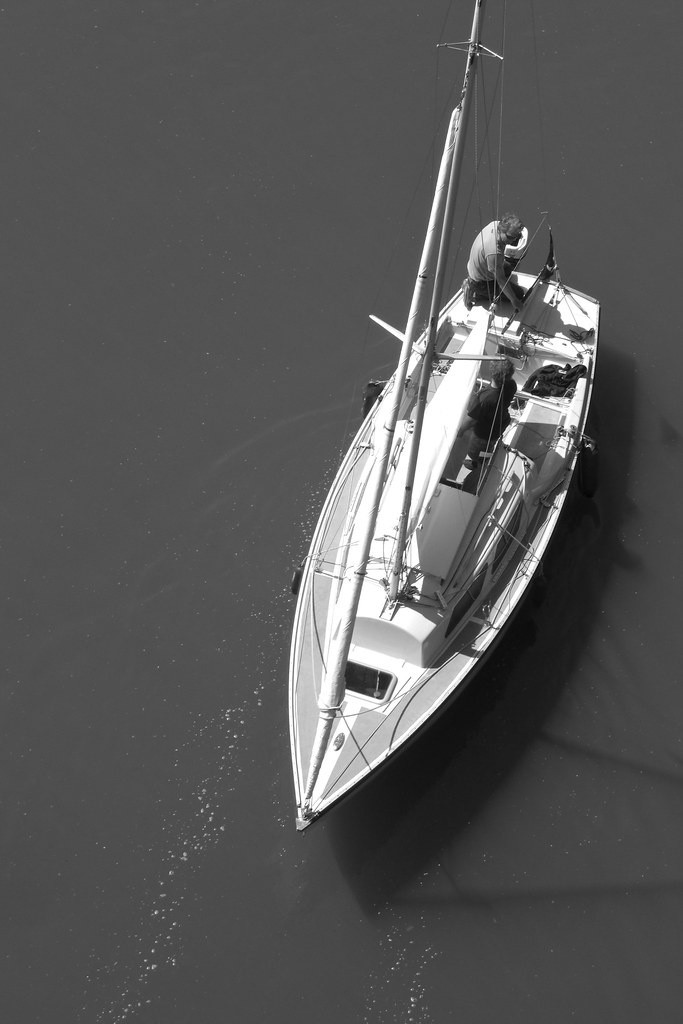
[462,278,474,310]
[463,459,474,469]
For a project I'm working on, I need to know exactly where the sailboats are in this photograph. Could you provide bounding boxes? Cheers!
[284,0,601,837]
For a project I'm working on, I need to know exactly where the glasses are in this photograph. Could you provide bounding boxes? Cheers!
[505,233,521,240]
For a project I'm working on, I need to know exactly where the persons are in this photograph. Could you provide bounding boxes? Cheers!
[462,211,524,310]
[458,354,517,470]
